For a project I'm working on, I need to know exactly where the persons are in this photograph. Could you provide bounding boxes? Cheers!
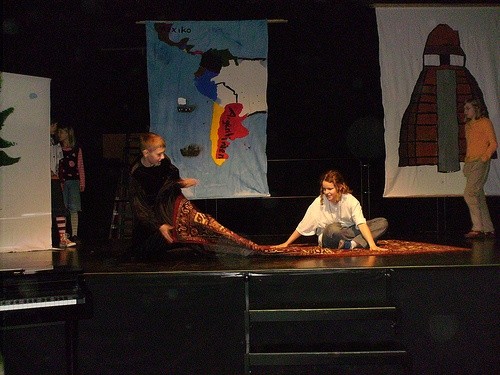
[126,132,197,258]
[270,172,389,252]
[50,118,86,248]
[462,99,497,238]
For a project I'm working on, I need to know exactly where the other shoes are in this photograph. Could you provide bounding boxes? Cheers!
[464,232,495,238]
[166,243,192,252]
[71,235,81,243]
[338,240,351,250]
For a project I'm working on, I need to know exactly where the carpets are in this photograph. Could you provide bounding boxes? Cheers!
[172,194,472,256]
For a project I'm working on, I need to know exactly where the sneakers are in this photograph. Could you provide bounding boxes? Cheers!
[60,233,76,248]
